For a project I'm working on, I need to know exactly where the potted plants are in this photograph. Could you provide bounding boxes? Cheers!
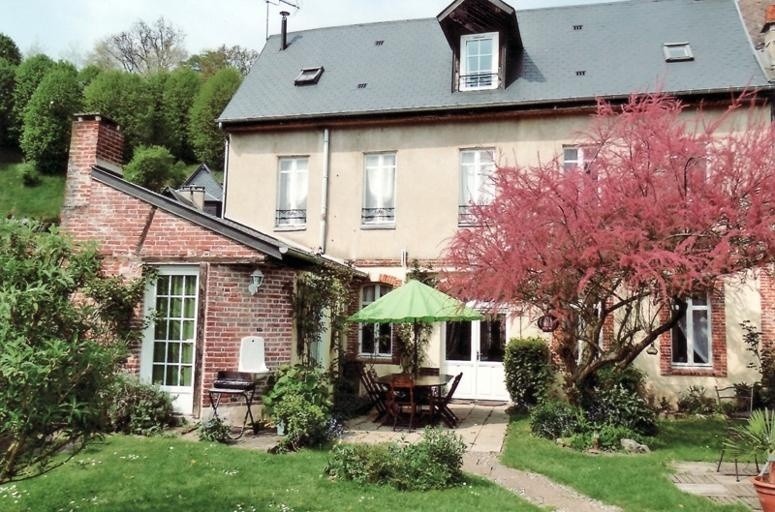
[722,407,775,512]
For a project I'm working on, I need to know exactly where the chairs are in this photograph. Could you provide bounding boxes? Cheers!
[358,367,463,430]
[715,385,760,483]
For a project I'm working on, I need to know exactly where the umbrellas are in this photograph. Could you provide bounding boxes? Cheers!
[346,278,487,378]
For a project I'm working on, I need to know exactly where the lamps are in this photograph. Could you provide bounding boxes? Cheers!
[248,267,264,295]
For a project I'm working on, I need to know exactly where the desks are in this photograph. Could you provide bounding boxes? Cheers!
[208,387,257,440]
